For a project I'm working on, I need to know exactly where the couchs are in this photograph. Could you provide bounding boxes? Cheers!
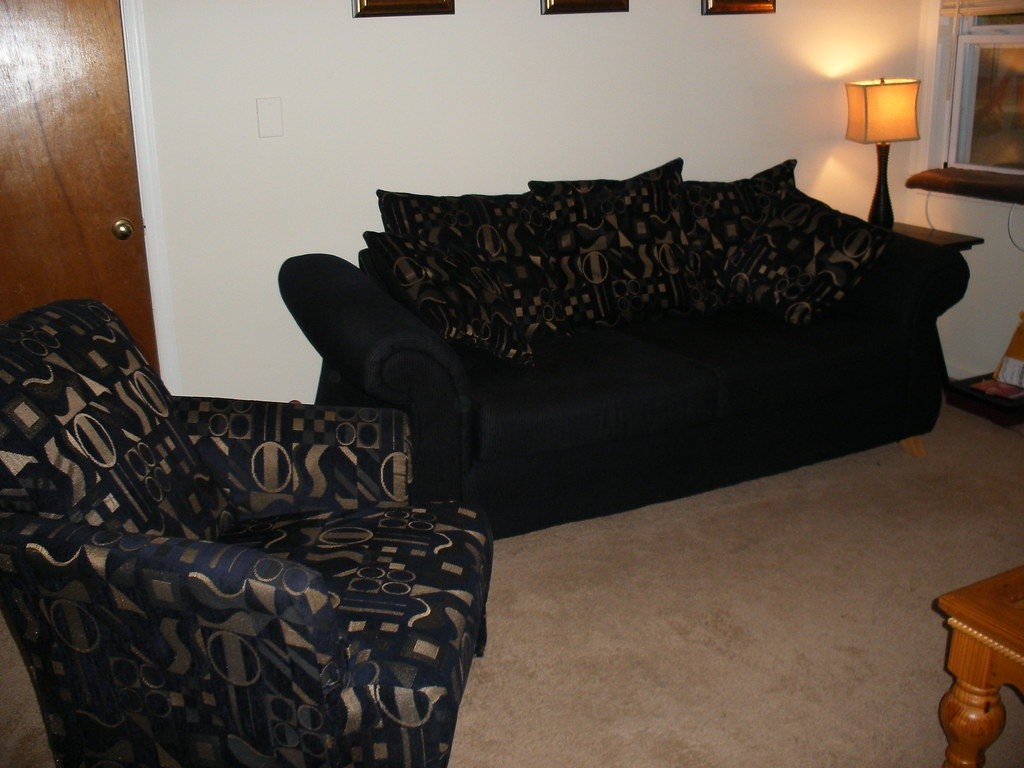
[277,184,971,540]
[0,297,494,768]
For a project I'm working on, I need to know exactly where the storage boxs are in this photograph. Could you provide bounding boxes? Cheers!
[946,311,1024,427]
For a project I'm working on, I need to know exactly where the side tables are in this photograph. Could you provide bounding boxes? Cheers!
[892,222,985,251]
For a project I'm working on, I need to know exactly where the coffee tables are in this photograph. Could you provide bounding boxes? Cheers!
[936,564,1024,768]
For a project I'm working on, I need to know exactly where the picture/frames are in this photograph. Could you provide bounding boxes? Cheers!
[350,0,455,19]
[701,0,776,15]
[540,0,629,15]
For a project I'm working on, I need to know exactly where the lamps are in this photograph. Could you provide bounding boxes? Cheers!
[845,77,921,228]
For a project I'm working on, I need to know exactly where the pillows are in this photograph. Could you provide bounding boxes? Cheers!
[362,157,893,368]
